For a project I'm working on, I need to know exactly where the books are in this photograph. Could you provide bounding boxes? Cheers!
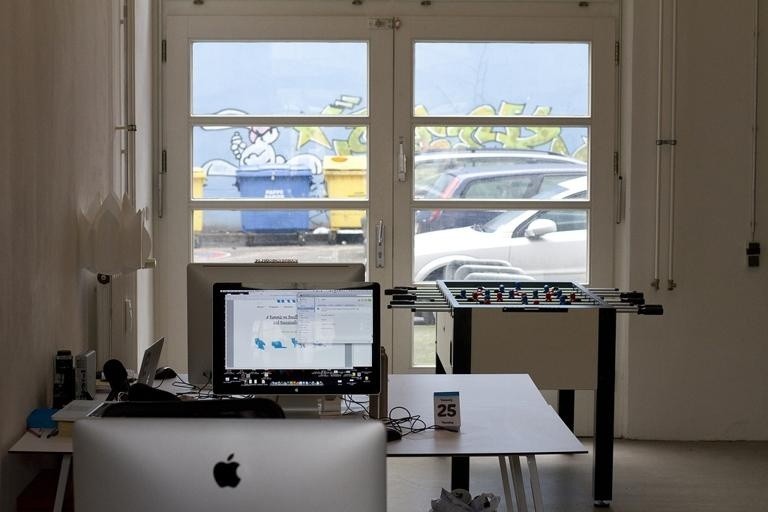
[51,399,104,422]
[57,403,102,438]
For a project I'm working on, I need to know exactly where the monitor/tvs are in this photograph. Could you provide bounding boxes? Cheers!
[185,261,366,388]
[211,281,383,419]
[71,415,389,512]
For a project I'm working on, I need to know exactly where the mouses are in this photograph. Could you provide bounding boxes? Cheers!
[153,365,177,379]
[386,426,402,442]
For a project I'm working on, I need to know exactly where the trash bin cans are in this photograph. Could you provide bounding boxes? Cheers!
[192,167,206,249]
[235,167,313,246]
[322,156,368,245]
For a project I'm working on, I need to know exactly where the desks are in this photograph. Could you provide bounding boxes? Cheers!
[8,373,588,512]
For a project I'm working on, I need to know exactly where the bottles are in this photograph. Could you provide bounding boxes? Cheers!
[368,346,389,419]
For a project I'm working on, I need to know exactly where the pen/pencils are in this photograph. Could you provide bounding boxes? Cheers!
[26,427,58,438]
